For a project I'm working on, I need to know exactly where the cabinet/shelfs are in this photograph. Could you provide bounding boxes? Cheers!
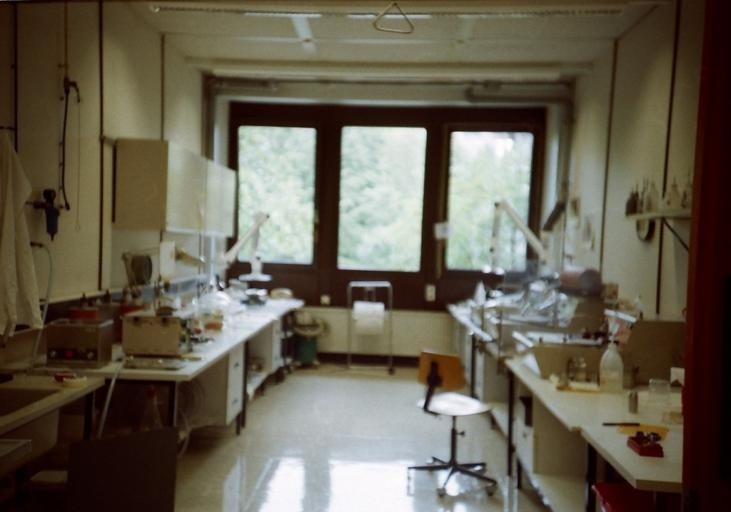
[205,158,238,238]
[114,137,207,234]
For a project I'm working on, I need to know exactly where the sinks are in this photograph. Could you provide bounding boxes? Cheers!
[0,375,108,477]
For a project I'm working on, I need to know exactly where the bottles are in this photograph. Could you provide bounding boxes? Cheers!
[599,343,624,393]
[625,177,692,215]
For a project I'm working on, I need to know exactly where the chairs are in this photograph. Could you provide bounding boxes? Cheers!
[76,425,179,512]
[407,351,498,497]
